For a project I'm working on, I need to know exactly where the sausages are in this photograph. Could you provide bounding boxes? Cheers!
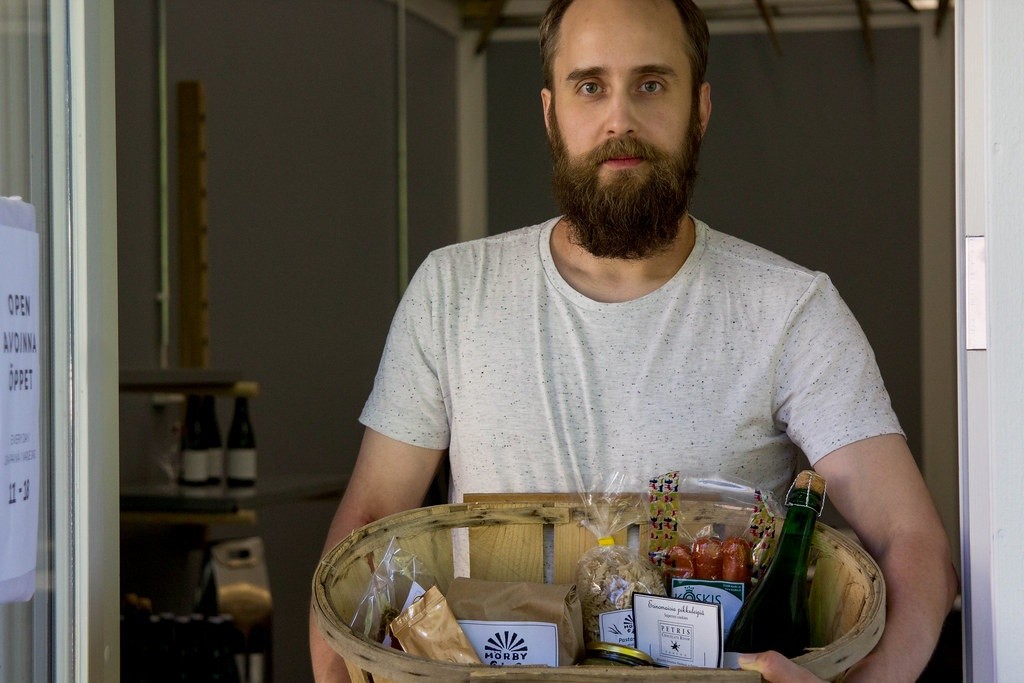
[657,536,751,593]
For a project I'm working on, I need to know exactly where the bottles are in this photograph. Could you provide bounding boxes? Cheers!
[200,394,222,485]
[223,396,260,486]
[722,471,824,671]
[178,396,207,487]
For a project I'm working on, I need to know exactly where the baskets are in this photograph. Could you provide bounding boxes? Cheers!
[313,492,887,683]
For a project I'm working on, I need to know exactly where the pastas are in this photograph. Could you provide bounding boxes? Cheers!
[576,555,666,641]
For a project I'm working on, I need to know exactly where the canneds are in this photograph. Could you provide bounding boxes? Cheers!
[579,642,653,666]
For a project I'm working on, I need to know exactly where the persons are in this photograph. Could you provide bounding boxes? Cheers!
[310,0,958,683]
